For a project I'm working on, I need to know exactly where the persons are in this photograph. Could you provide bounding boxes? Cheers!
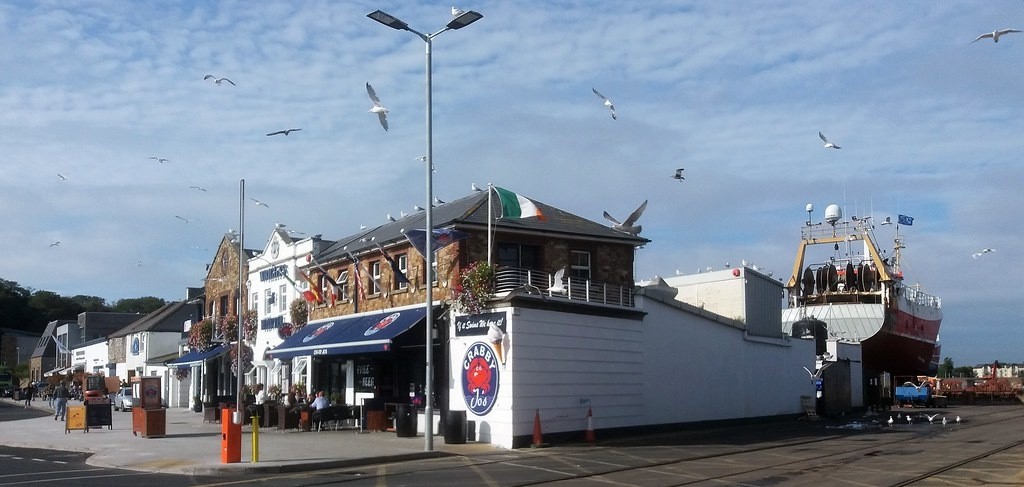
[255,383,268,428]
[293,388,329,431]
[24,379,83,421]
[122,379,126,387]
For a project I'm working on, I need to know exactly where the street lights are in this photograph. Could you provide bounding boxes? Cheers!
[367,6,485,452]
[16,346,20,365]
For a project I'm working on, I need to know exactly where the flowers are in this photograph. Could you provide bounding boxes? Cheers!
[221,312,239,348]
[289,297,308,330]
[289,383,306,405]
[229,341,253,377]
[451,260,497,320]
[267,385,282,401]
[172,368,191,382]
[242,309,257,345]
[187,319,212,353]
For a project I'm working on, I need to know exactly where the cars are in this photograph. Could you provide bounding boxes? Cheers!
[36,382,48,393]
[114,386,133,411]
[4,385,17,397]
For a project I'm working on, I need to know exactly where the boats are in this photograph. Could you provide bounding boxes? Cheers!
[783,203,942,404]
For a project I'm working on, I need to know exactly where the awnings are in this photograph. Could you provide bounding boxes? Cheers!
[267,304,438,357]
[167,344,233,365]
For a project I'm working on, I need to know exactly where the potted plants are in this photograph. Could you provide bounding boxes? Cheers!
[194,394,202,412]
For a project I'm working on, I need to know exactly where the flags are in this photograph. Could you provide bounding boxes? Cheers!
[404,228,471,260]
[898,214,914,225]
[353,256,366,300]
[315,261,339,308]
[281,272,317,304]
[379,244,410,284]
[299,270,324,302]
[492,186,544,222]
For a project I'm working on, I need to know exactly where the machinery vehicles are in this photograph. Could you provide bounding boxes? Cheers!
[83,375,109,405]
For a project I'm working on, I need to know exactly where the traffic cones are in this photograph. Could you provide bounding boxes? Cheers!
[582,409,597,442]
[529,408,549,447]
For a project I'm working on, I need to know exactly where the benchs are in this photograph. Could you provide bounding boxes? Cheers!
[312,404,353,432]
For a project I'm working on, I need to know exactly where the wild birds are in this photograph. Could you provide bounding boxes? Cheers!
[887,380,962,428]
[593,87,616,120]
[971,248,994,258]
[803,362,833,383]
[49,241,60,246]
[501,265,567,303]
[603,199,647,236]
[452,6,464,17]
[58,174,65,181]
[970,29,1023,44]
[819,132,841,149]
[204,74,236,86]
[675,260,774,281]
[177,185,206,224]
[671,168,684,182]
[366,81,390,132]
[266,129,301,137]
[359,156,483,233]
[223,229,240,245]
[250,198,295,234]
[151,156,167,164]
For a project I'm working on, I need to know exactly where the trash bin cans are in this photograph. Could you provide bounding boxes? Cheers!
[366,399,386,430]
